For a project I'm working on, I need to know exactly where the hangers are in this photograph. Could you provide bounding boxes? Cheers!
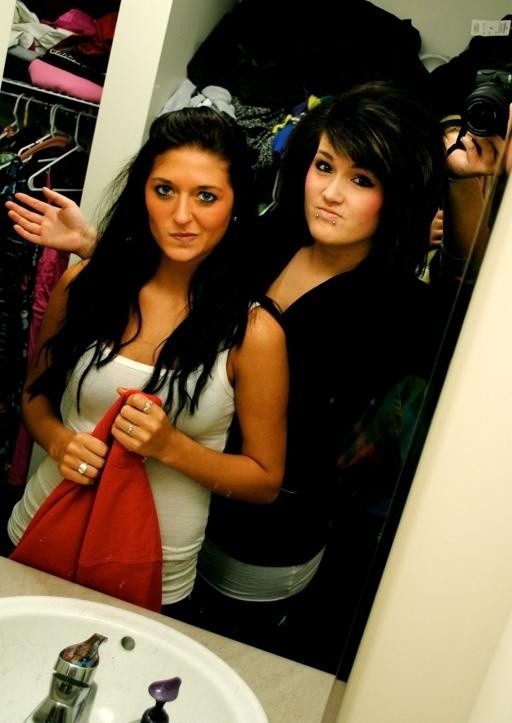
[0,93,89,192]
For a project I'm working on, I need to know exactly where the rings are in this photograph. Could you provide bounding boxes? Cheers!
[143,401,154,412]
[127,426,133,434]
[77,462,89,475]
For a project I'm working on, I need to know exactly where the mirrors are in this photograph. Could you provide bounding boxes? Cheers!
[0,0,512,677]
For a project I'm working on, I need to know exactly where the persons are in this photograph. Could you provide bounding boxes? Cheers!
[4,81,511,655]
[1,106,289,615]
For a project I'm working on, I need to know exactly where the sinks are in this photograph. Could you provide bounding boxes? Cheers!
[0,596,273,723]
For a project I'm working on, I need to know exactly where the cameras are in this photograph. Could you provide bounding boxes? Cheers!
[462,67,512,137]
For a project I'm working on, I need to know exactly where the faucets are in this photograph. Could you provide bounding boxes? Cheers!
[17,631,107,722]
